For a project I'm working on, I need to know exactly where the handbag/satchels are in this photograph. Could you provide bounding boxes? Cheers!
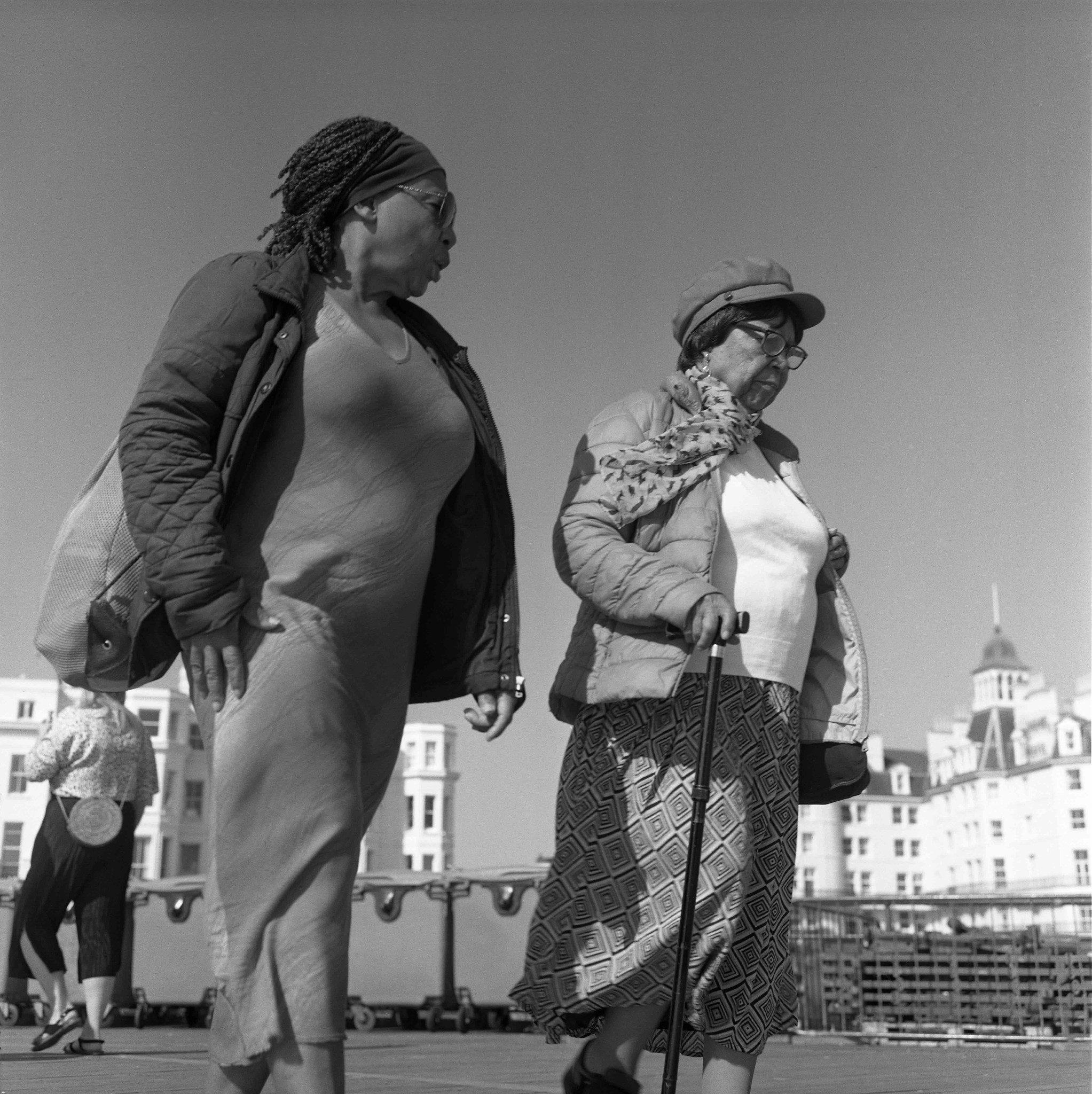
[797,741,870,805]
[39,421,180,693]
[67,795,125,845]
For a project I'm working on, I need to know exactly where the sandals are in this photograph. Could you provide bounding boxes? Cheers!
[30,1006,82,1053]
[63,1035,104,1053]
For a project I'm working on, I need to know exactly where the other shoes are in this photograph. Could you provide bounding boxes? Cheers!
[561,1039,642,1094]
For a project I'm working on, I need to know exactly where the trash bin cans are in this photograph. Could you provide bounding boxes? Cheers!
[453,860,557,1033]
[0,891,121,1027]
[350,868,452,1030]
[126,874,220,1031]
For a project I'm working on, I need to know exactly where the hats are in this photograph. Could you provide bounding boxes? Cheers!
[672,254,826,350]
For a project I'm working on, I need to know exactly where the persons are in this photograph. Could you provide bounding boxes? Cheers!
[120,116,526,1094]
[509,251,869,1094]
[6,679,158,1057]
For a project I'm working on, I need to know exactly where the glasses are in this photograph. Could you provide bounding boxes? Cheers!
[395,184,457,230]
[734,320,808,370]
